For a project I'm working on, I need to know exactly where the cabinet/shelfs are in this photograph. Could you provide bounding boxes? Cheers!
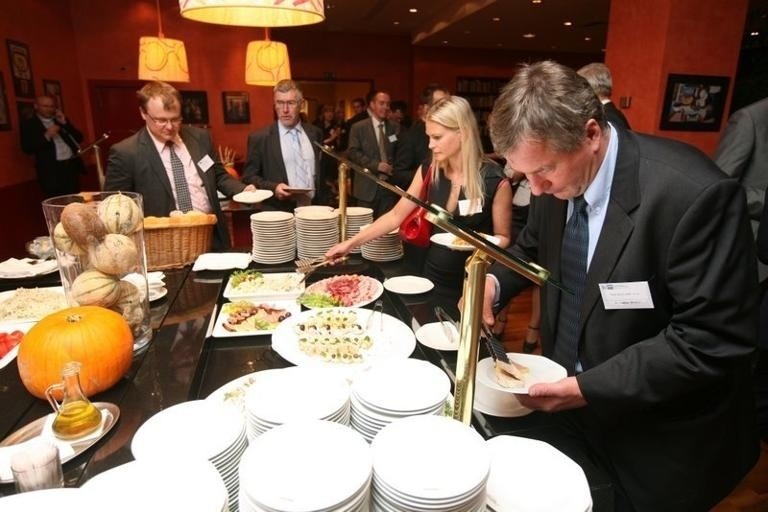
[456,75,516,156]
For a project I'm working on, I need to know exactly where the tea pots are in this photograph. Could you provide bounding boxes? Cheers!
[45,362,100,442]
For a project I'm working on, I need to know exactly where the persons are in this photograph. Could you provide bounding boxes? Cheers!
[711,94,767,288]
[449,58,767,512]
[103,80,256,253]
[21,95,84,197]
[237,77,338,213]
[574,61,630,130]
[229,85,451,225]
[319,92,514,305]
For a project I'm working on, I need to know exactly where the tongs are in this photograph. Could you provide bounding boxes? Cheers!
[365,299,384,331]
[433,306,460,342]
[482,324,521,382]
[295,253,350,275]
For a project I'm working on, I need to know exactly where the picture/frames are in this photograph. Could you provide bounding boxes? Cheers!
[223,91,253,124]
[6,39,37,100]
[42,80,66,113]
[658,74,730,133]
[0,72,13,130]
[176,88,210,125]
[17,101,39,155]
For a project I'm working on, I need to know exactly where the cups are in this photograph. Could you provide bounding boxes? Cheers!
[11,443,64,493]
[42,190,154,355]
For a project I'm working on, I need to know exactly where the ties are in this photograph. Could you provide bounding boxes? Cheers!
[377,124,388,180]
[288,127,310,207]
[163,140,193,214]
[551,192,589,376]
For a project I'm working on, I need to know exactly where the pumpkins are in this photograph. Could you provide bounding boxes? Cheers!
[54,194,143,337]
[17,305,134,402]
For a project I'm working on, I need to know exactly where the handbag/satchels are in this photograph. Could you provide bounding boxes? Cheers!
[399,163,432,248]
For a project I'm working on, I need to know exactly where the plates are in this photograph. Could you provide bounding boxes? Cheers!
[416,321,460,352]
[474,352,568,396]
[303,274,386,309]
[383,275,435,295]
[0,256,76,370]
[251,205,405,264]
[244,366,350,443]
[349,358,451,447]
[237,419,373,512]
[283,188,317,194]
[471,383,534,418]
[367,416,490,511]
[232,189,274,204]
[1,400,118,483]
[430,232,500,252]
[212,272,306,339]
[485,435,593,512]
[148,270,168,302]
[131,399,248,512]
[269,305,417,365]
[77,454,232,511]
[0,486,78,512]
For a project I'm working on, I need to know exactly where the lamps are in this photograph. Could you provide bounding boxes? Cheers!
[178,1,328,31]
[134,1,191,84]
[244,27,293,88]
